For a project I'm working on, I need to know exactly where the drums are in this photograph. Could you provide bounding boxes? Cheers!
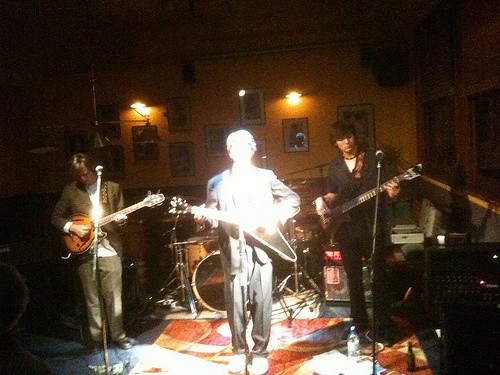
[184,236,216,269]
[293,225,320,241]
[192,250,227,314]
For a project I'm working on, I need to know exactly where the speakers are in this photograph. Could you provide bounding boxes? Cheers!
[145,215,192,302]
[74,259,139,323]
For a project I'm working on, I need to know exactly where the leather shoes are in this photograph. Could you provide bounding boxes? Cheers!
[113,336,132,350]
[90,342,103,354]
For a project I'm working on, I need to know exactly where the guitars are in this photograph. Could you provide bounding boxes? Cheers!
[319,158,427,246]
[171,198,301,263]
[60,192,163,256]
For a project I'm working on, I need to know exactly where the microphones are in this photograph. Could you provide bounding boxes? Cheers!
[95,165,103,178]
[376,150,384,163]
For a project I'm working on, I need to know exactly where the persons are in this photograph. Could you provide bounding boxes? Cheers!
[50,152,132,350]
[315,121,402,346]
[0,262,58,375]
[290,133,305,148]
[194,129,301,374]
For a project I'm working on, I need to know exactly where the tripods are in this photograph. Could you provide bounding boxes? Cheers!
[272,223,322,297]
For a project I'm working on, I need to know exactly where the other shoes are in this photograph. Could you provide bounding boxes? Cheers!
[227,354,247,374]
[251,357,269,375]
[379,327,399,348]
[355,327,370,345]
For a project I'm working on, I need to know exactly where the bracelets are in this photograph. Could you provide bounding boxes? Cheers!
[199,224,203,228]
[321,196,326,201]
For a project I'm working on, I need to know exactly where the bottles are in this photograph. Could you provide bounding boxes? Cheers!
[407,341,415,371]
[347,326,360,364]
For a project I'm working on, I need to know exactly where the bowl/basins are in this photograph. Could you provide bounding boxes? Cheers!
[437,236,445,245]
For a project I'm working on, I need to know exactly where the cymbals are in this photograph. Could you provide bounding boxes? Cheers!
[158,213,197,223]
[279,181,322,189]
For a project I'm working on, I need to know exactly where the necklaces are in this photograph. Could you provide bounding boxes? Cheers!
[343,155,355,160]
